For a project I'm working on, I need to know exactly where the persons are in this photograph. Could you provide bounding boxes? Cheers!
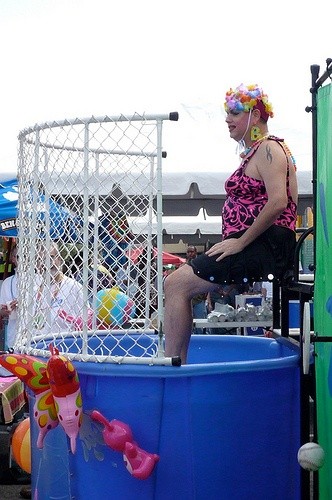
[164,85,298,358]
[91,259,265,318]
[0,242,90,349]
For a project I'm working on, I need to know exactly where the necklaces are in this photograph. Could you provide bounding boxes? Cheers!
[240,135,268,158]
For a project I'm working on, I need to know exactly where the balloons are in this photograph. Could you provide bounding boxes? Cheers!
[92,410,159,480]
[0,343,83,452]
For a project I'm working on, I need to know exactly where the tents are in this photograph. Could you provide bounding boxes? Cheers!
[0,173,81,239]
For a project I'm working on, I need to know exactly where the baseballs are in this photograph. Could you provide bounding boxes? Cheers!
[297,442,325,472]
